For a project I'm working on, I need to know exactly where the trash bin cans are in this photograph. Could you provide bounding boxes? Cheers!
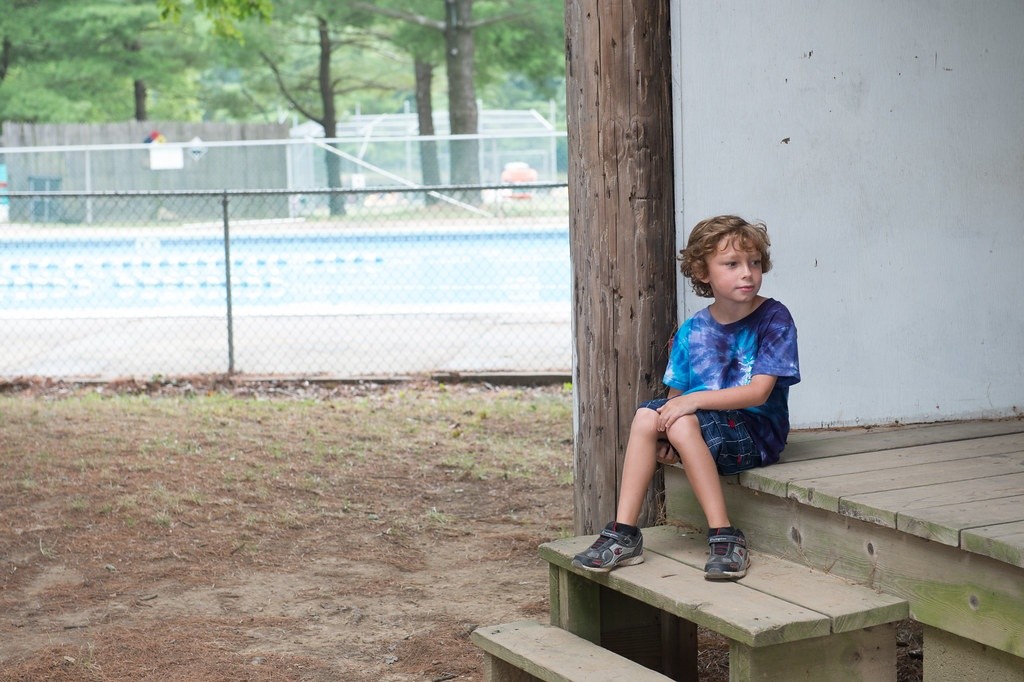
[501,162,539,216]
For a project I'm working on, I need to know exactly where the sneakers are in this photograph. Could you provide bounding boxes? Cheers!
[571,521,644,572]
[703,527,752,580]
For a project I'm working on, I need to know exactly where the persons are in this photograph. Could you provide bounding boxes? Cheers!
[571,214,801,581]
